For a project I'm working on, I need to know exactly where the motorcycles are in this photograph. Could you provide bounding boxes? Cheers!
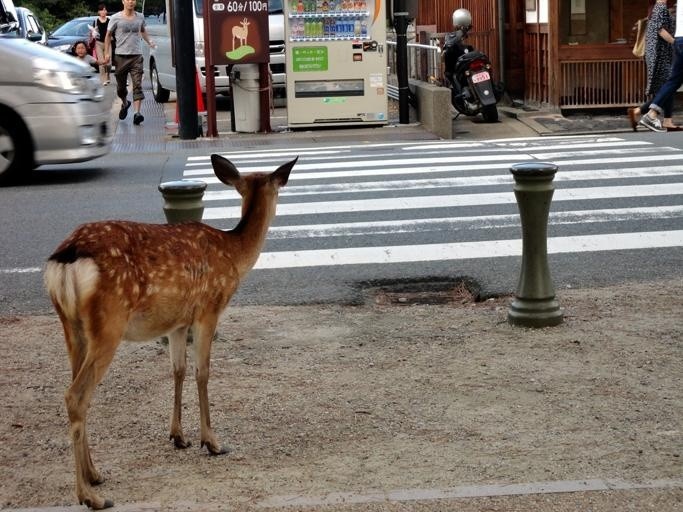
[441,24,506,123]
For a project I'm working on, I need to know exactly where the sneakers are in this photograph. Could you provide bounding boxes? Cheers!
[103,79,110,86]
[133,112,144,125]
[119,101,131,120]
[628,107,638,131]
[639,114,667,133]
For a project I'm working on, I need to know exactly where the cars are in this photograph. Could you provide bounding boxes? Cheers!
[0,32,113,188]
[47,15,111,56]
[0,0,48,46]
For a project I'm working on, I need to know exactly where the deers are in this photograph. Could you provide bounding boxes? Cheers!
[43,154,299,510]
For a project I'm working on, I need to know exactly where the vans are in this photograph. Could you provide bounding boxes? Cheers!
[141,0,286,111]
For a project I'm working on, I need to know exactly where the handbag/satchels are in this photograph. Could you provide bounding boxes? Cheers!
[88,31,96,48]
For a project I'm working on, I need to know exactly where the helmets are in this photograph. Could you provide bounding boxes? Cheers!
[452,8,472,26]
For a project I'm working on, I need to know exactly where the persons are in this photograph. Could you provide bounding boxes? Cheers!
[626,0,683,131]
[104,1,157,125]
[638,0,682,133]
[70,41,107,68]
[93,4,112,87]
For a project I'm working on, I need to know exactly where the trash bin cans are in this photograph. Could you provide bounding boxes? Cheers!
[230,63,260,132]
